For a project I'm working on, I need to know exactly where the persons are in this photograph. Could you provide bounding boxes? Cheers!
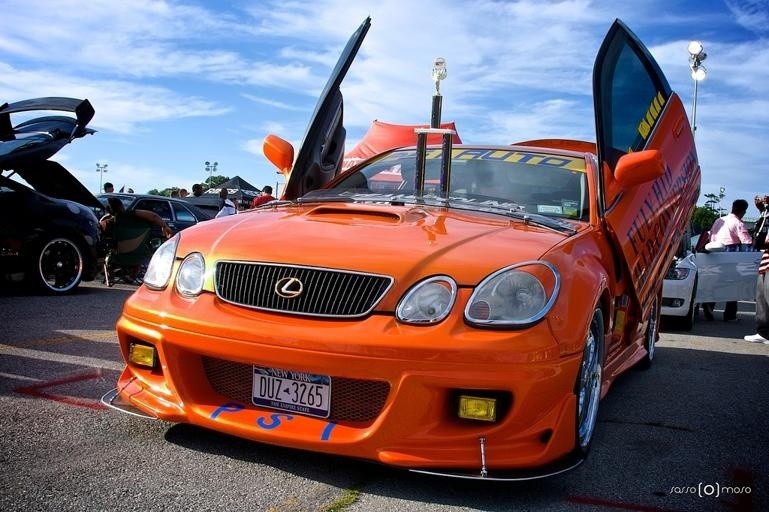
[699,194,769,347]
[97,181,276,281]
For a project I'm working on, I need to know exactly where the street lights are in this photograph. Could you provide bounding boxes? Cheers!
[688,41,707,140]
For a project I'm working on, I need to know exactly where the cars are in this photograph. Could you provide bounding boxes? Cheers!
[0,95,764,333]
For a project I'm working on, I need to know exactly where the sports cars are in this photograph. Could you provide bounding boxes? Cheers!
[99,16,702,482]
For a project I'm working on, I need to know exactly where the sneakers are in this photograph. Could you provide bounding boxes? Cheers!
[744,333,769,345]
[703,303,715,321]
